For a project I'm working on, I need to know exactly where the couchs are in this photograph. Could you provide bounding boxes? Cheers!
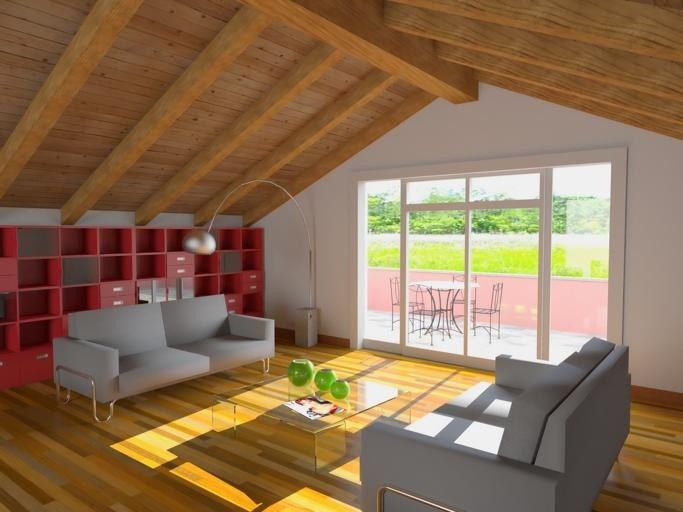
[52,292,274,423]
[361,338,633,512]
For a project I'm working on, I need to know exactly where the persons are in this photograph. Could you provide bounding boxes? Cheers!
[294,397,336,418]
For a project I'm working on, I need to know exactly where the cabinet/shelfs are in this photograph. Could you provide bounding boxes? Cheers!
[0,225,263,389]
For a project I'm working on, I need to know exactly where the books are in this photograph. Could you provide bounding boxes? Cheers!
[281,395,344,421]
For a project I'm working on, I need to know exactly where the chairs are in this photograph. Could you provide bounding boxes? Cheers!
[469,283,503,344]
[450,273,477,326]
[390,277,424,333]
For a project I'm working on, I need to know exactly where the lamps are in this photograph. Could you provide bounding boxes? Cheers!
[183,179,319,350]
